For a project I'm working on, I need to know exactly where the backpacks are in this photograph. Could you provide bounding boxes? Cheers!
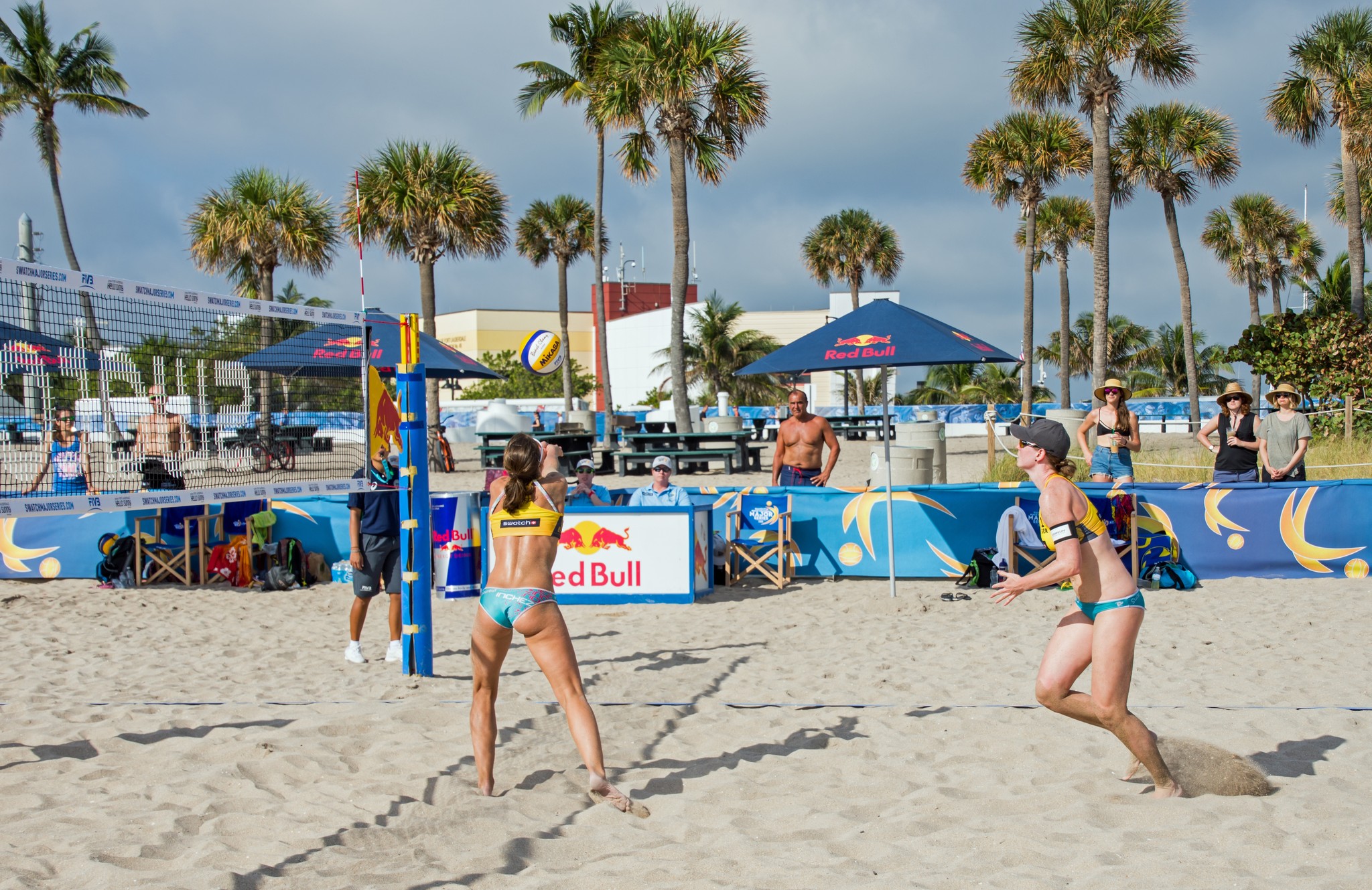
[261,537,332,592]
[96,533,147,585]
[1138,533,1197,590]
[712,533,733,586]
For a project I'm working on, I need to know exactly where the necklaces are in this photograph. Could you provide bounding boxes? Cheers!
[1237,414,1243,424]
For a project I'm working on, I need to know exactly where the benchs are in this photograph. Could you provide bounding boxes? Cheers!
[610,450,736,477]
[829,426,881,441]
[561,448,620,476]
[744,428,778,441]
[111,425,333,457]
[647,445,768,470]
[472,447,505,467]
[0,430,23,443]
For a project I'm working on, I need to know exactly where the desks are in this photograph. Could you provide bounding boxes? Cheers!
[619,432,758,471]
[235,427,257,438]
[281,425,317,436]
[188,426,224,439]
[474,432,555,468]
[743,417,780,442]
[529,433,601,472]
[0,422,24,431]
[825,414,898,441]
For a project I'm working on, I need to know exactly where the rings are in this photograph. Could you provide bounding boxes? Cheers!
[1119,437,1121,440]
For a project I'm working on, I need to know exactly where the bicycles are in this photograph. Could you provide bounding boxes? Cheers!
[427,415,456,472]
[224,426,297,474]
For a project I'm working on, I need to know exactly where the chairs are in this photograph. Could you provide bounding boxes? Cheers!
[134,504,209,587]
[722,492,791,590]
[1006,494,1138,592]
[197,497,272,587]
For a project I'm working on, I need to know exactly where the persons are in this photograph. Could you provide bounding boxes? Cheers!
[772,389,840,488]
[133,384,196,490]
[1253,383,1313,483]
[566,459,611,506]
[733,405,740,417]
[1077,379,1141,483]
[470,431,634,814]
[280,407,288,426]
[22,406,107,498]
[699,404,708,422]
[1196,383,1262,483]
[344,442,404,663]
[991,419,1184,799]
[627,456,692,506]
[556,410,563,424]
[531,412,543,431]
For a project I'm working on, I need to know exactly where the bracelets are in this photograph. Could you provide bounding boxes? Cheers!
[351,549,360,553]
[1124,438,1127,446]
[350,546,359,549]
[1050,520,1079,545]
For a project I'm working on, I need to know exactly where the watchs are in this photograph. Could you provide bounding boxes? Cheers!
[588,489,595,497]
[772,405,780,424]
[1209,445,1215,452]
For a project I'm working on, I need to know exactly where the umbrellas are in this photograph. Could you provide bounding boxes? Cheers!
[240,308,504,380]
[733,297,1026,599]
[0,320,141,375]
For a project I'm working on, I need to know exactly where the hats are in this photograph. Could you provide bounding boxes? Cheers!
[774,406,779,408]
[1094,378,1132,403]
[653,456,672,469]
[1009,419,1070,459]
[557,411,562,414]
[732,405,738,408]
[1217,382,1301,407]
[576,459,595,472]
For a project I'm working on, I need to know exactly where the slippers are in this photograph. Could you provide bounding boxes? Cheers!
[90,581,114,588]
[1056,581,1073,591]
[940,593,968,601]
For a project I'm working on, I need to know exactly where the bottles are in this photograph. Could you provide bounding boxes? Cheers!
[1136,578,1151,588]
[998,558,1008,588]
[119,572,128,588]
[990,566,998,588]
[331,559,354,584]
[1151,567,1161,589]
[126,567,136,587]
[111,578,125,588]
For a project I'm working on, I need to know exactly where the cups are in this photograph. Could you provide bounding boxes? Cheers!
[1226,427,1236,441]
[1108,435,1119,454]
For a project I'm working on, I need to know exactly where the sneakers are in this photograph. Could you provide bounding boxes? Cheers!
[345,643,404,663]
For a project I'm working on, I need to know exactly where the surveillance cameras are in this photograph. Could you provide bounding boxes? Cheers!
[631,262,636,267]
[604,267,608,271]
[695,275,698,279]
[1044,375,1047,379]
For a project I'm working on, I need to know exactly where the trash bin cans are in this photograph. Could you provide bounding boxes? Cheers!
[569,410,596,447]
[1045,409,1089,451]
[917,410,936,420]
[896,421,946,484]
[870,445,935,487]
[702,416,743,472]
[433,492,476,596]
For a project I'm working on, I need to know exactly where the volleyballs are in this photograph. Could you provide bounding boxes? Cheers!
[519,329,565,377]
[98,533,120,556]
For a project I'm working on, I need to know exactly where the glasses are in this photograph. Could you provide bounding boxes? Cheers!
[149,394,168,401]
[1224,395,1240,403]
[1019,439,1054,452]
[532,437,543,468]
[577,468,594,474]
[1275,392,1290,398]
[1104,388,1119,395]
[653,466,671,472]
[58,415,76,423]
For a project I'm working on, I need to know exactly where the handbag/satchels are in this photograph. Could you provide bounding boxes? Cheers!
[533,418,544,431]
[955,546,999,589]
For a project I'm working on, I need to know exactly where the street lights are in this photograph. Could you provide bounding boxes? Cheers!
[620,242,637,311]
